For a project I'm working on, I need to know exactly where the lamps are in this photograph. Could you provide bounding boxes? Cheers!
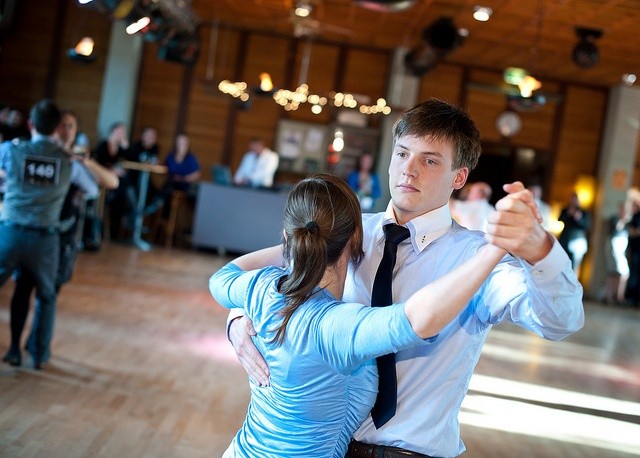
[99,0,198,67]
[571,26,603,70]
[404,16,465,78]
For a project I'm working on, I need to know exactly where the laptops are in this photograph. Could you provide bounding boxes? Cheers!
[212,165,235,186]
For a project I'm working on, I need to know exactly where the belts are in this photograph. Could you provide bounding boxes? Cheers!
[348,439,431,458]
[6,222,56,235]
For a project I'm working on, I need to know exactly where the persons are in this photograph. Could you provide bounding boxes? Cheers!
[0,100,100,370]
[557,191,592,270]
[0,107,162,251]
[346,152,382,210]
[207,173,543,458]
[233,135,280,187]
[227,99,586,458]
[155,132,200,239]
[450,182,496,232]
[606,189,637,307]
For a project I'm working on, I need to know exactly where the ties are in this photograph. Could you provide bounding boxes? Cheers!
[368,222,410,430]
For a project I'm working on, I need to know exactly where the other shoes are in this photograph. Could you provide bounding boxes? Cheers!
[33,359,46,369]
[3,350,22,366]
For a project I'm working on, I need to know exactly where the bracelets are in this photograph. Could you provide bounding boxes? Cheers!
[227,315,244,346]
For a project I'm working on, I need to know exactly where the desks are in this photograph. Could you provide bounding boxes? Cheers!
[190,182,289,257]
[122,160,169,252]
